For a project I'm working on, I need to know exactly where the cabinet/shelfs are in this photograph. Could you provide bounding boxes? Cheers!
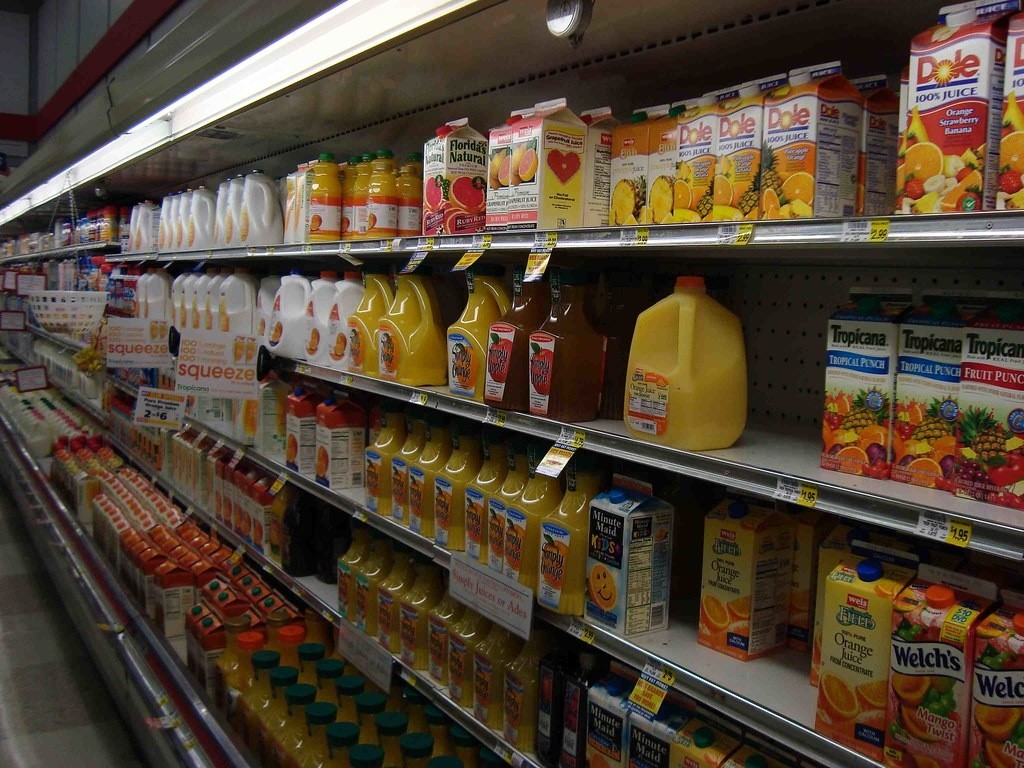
[0,0,1024,768]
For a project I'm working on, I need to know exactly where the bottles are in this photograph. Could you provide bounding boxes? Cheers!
[623,273,749,450]
[0,150,602,768]
